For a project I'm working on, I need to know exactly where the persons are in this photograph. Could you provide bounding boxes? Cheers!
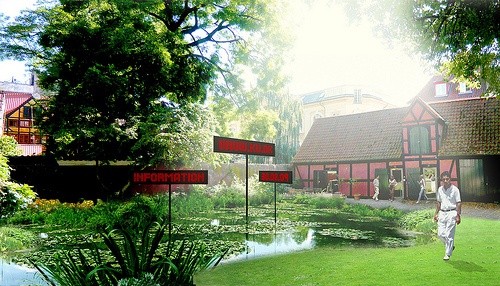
[388,175,398,202]
[416,173,431,204]
[434,171,461,262]
[372,175,380,201]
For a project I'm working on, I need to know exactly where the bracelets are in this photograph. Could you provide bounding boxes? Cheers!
[458,213,461,216]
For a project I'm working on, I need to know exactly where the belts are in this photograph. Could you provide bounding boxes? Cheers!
[440,209,454,212]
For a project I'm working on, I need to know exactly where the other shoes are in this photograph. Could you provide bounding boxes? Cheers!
[443,255,450,260]
